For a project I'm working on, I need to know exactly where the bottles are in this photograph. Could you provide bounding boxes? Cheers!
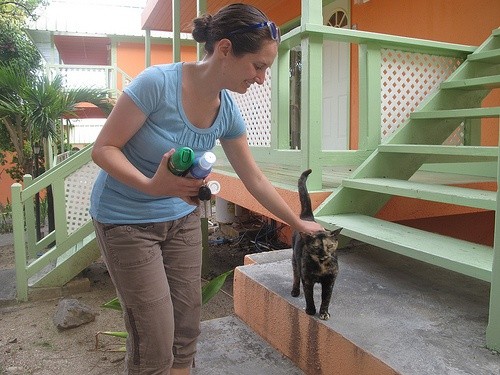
[168,147,194,175]
[183,152,216,180]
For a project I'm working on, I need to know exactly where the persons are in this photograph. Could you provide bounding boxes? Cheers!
[89,3,326,375]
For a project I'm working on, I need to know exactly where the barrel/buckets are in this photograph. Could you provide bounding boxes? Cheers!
[216,196,235,222]
[235,204,249,216]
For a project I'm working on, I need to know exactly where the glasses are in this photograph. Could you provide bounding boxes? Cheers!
[223,20,281,45]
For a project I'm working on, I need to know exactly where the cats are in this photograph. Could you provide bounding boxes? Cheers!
[291,169,344,320]
